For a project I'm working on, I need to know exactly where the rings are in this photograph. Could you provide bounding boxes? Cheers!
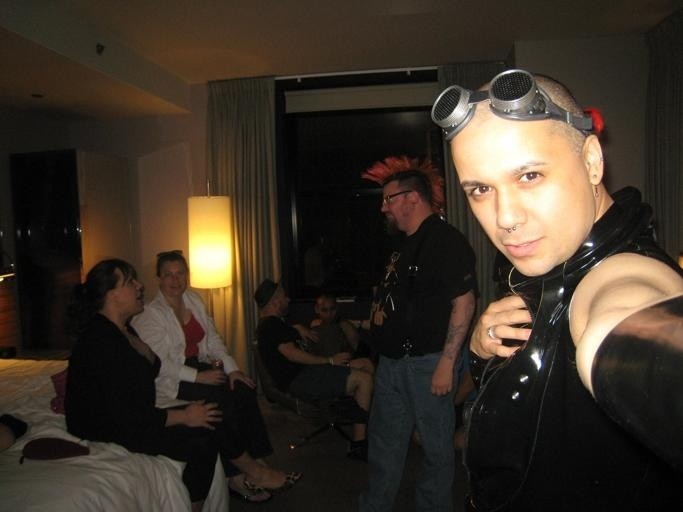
[486,325,500,340]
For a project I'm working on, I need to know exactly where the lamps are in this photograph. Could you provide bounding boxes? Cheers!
[187,180,234,320]
[0,250,16,278]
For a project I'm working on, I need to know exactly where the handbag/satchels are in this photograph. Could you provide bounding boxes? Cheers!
[24,437,89,460]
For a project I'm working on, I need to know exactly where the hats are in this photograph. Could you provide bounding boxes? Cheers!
[254,278,278,308]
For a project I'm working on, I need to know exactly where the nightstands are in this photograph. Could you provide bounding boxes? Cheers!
[0,282,21,358]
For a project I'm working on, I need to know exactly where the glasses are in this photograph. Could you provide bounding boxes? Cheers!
[429,68,593,142]
[383,191,412,208]
[319,305,336,311]
[155,249,182,257]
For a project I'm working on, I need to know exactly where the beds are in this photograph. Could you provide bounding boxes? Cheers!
[0,359,231,512]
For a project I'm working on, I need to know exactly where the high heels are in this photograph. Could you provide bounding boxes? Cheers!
[229,481,273,503]
[243,471,303,496]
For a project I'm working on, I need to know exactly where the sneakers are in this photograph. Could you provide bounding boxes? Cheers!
[346,439,367,459]
[330,400,369,422]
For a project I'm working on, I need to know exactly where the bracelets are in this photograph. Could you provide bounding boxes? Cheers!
[329,355,334,366]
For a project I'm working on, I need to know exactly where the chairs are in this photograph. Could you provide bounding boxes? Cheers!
[250,348,364,454]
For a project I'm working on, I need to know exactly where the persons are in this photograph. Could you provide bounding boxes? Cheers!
[61,257,305,512]
[428,68,683,511]
[127,250,278,469]
[253,278,376,461]
[361,157,476,512]
[309,293,364,360]
